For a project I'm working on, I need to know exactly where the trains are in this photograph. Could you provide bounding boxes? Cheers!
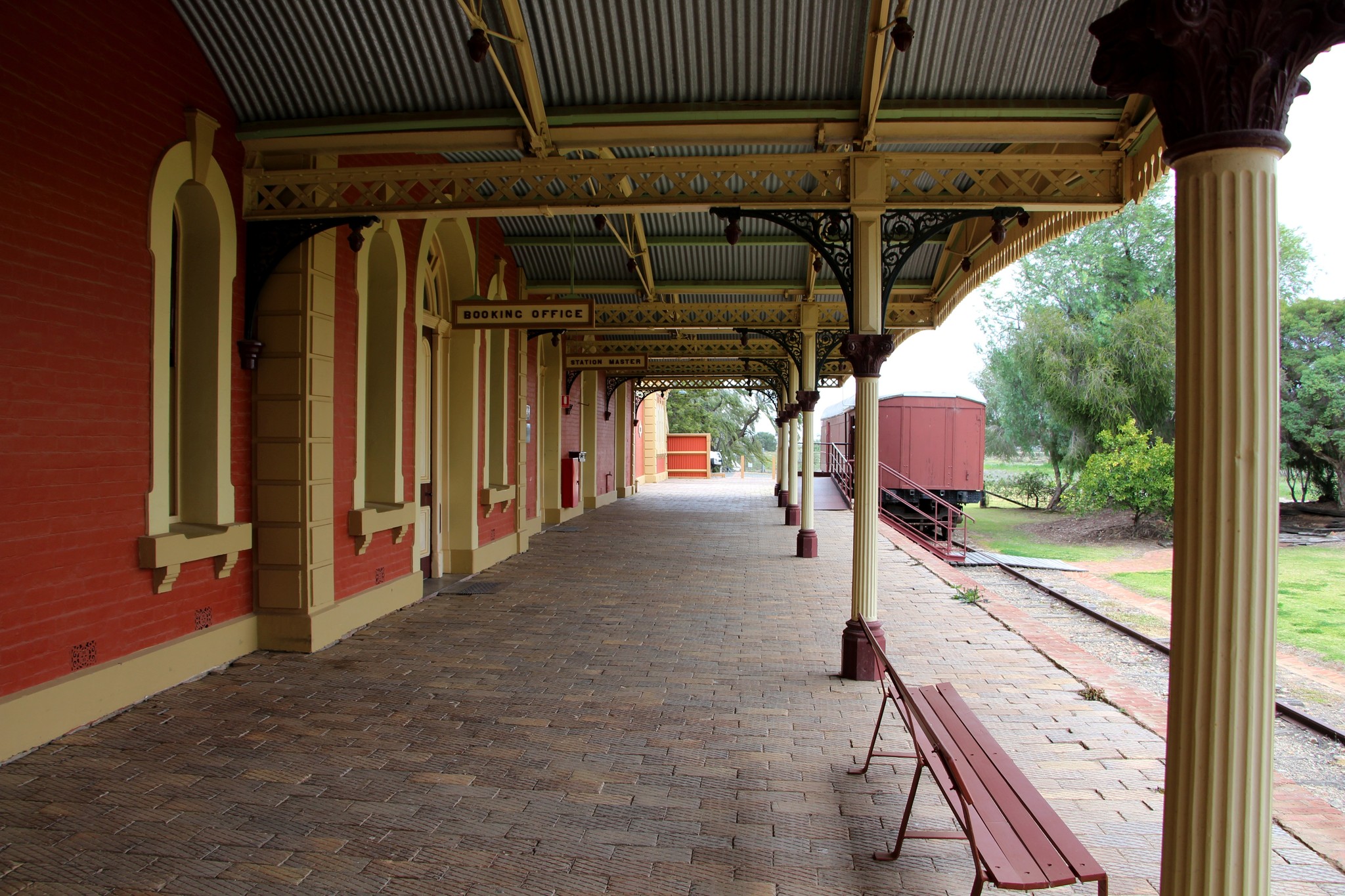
[821,396,987,540]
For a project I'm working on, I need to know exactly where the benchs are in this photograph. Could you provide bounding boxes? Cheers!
[844,611,1109,896]
